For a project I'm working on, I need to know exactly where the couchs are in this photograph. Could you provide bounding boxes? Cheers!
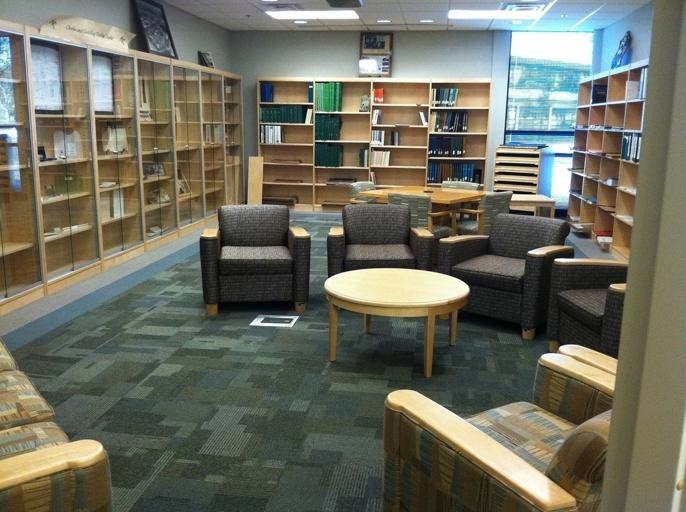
[0,335,113,512]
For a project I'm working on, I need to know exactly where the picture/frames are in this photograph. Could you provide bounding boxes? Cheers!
[358,31,394,76]
[130,0,179,59]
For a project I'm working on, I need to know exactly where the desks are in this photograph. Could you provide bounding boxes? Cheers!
[508,194,556,219]
[357,188,488,235]
[323,268,471,379]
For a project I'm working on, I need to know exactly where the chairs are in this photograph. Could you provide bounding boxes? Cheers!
[383,352,621,512]
[558,344,617,375]
[549,258,628,358]
[326,203,435,311]
[438,212,574,341]
[456,191,514,235]
[198,203,311,318]
[387,193,453,239]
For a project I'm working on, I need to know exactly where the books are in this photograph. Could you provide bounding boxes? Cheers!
[568,66,650,244]
[260,82,476,185]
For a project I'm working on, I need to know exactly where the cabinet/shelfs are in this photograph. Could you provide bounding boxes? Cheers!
[137,58,179,253]
[30,36,101,297]
[173,64,205,237]
[1,28,42,313]
[492,145,541,216]
[223,75,247,206]
[200,70,226,227]
[91,49,143,273]
[566,57,649,262]
[254,76,492,213]
